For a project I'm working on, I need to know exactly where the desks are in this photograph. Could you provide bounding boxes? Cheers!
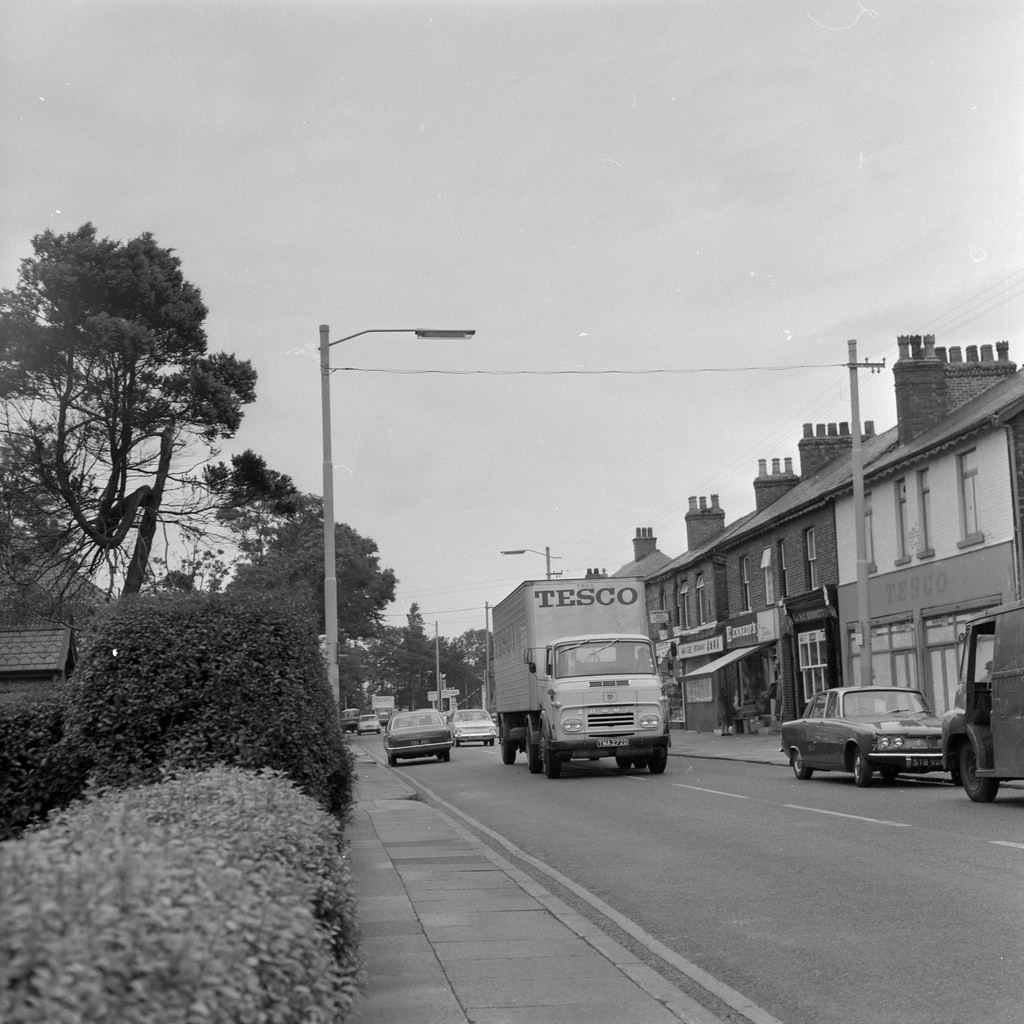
[732,716,759,736]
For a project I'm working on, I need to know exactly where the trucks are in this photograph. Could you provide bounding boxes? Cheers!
[341,709,360,733]
[372,696,395,725]
[492,577,677,779]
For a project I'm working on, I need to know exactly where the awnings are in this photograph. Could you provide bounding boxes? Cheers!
[683,641,773,680]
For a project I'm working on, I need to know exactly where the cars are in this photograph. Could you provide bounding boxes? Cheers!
[382,706,496,766]
[778,686,943,788]
[357,715,381,735]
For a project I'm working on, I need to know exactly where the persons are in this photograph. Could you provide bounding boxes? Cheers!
[770,675,783,721]
[717,689,734,736]
[979,660,993,682]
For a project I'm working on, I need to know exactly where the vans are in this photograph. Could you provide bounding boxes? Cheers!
[940,598,1024,801]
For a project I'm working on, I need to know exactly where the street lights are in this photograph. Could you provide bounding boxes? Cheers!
[318,323,476,730]
[501,546,562,579]
[419,621,441,712]
